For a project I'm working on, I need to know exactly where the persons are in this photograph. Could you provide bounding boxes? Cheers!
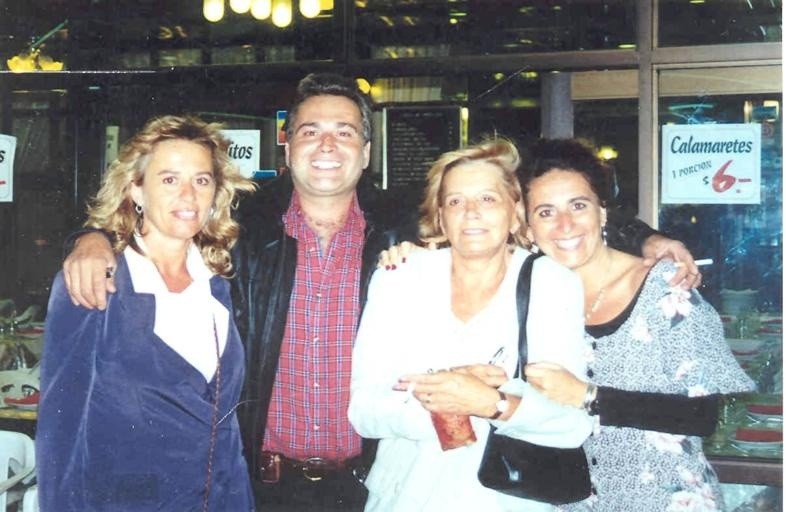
[63,71,703,512]
[375,138,757,512]
[34,115,256,512]
[347,128,594,512]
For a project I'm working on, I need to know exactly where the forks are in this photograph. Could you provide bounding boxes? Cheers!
[746,414,783,423]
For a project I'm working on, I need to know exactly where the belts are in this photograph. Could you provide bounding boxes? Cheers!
[260,450,363,480]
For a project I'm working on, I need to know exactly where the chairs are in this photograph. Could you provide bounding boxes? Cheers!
[0,430,38,512]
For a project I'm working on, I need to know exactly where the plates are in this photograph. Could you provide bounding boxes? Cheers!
[4,395,38,411]
[726,424,782,455]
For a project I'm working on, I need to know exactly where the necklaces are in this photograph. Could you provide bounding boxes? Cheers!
[305,214,346,230]
[585,288,605,319]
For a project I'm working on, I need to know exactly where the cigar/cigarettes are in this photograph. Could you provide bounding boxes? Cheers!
[404,383,416,404]
[674,259,713,267]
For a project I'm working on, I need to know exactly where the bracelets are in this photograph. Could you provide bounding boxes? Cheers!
[584,384,596,408]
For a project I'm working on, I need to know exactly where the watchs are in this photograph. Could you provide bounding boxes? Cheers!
[490,390,509,420]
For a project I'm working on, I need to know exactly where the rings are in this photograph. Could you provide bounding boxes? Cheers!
[426,393,431,403]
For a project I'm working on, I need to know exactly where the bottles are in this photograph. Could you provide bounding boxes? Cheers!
[421,369,479,454]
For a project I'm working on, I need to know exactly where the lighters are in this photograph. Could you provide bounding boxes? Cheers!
[106,266,113,278]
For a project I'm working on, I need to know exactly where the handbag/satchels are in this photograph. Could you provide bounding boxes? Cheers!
[475,252,594,507]
